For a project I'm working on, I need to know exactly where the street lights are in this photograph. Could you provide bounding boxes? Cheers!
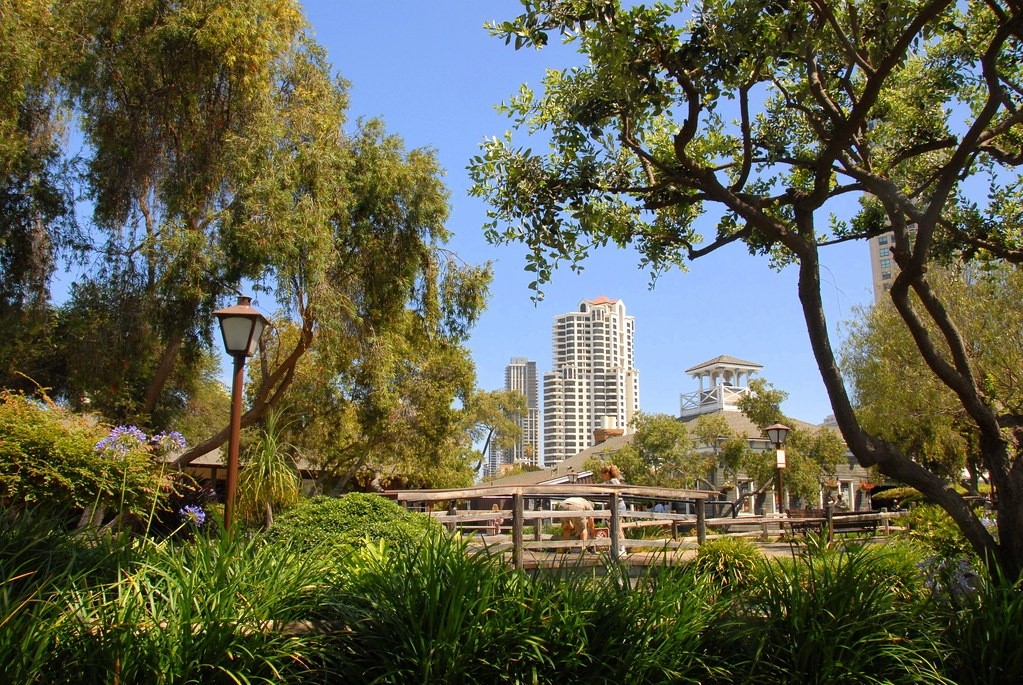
[213,295,276,531]
[765,419,791,536]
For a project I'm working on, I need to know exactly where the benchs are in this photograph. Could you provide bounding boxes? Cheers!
[826,510,880,544]
[785,508,826,539]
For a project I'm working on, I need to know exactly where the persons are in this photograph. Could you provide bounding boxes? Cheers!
[834,494,847,507]
[559,497,596,555]
[645,502,668,512]
[601,464,628,556]
[891,499,901,510]
[983,492,999,509]
